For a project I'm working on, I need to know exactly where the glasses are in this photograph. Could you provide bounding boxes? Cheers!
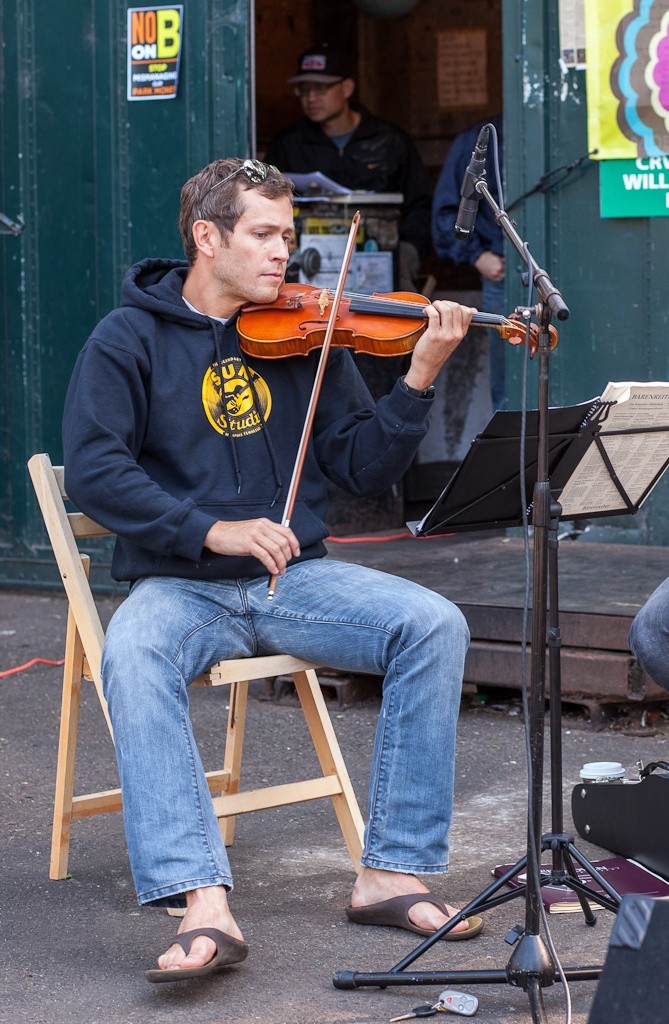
[198,159,282,221]
[294,78,344,97]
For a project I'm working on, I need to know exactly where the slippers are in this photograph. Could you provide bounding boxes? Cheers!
[144,927,249,983]
[344,891,485,942]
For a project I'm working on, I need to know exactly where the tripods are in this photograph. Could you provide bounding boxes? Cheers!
[332,187,627,1023]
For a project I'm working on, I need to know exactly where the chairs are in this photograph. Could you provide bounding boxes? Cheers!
[27,453,366,880]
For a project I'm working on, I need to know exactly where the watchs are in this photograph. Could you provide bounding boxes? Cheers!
[400,375,435,399]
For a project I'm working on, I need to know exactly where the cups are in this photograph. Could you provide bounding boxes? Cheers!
[579,761,627,784]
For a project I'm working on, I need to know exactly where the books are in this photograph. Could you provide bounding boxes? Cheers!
[491,856,669,914]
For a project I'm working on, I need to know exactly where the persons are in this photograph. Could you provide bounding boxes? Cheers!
[60,156,487,983]
[430,113,504,414]
[258,41,429,294]
[627,576,669,691]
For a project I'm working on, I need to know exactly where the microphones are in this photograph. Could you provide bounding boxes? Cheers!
[456,123,492,238]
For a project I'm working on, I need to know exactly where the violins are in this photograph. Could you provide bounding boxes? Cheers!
[235,280,557,361]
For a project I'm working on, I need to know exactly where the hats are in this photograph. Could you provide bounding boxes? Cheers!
[289,47,352,83]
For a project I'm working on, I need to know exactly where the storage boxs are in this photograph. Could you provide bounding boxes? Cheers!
[293,192,404,249]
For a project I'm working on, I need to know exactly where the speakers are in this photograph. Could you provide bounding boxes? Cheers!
[585,898,669,1023]
